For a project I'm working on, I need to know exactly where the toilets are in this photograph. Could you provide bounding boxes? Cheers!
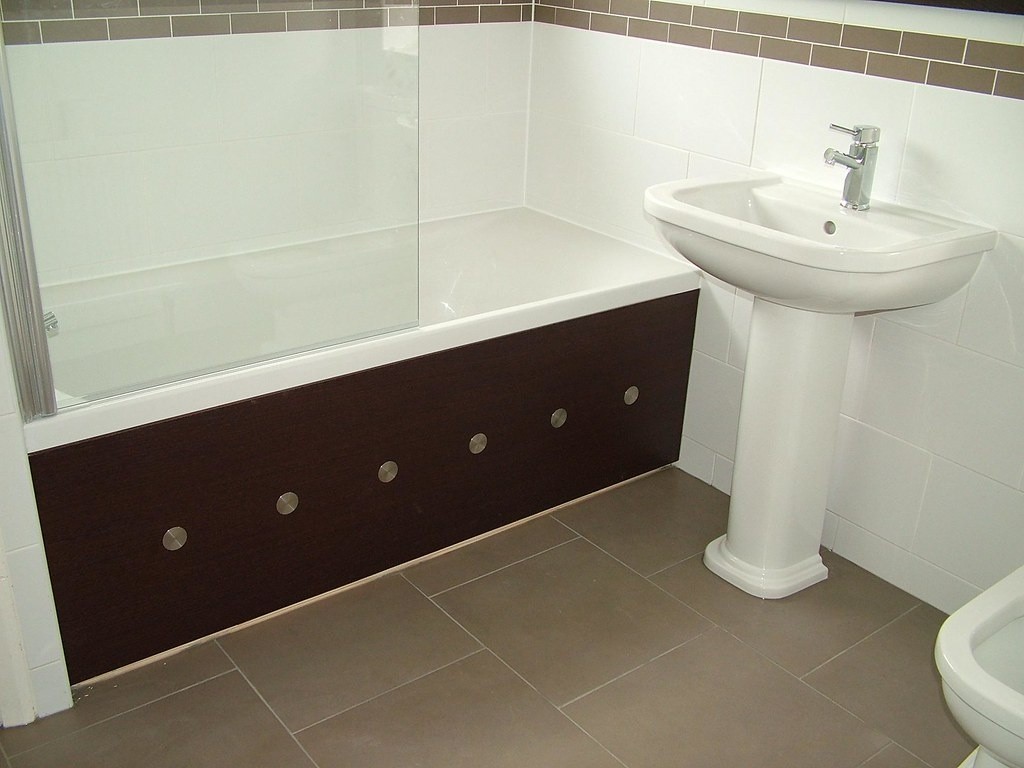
[934,563,1024,767]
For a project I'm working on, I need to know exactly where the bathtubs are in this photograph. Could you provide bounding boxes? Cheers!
[24,206,701,686]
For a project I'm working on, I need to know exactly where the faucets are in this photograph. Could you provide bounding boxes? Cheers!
[823,119,879,214]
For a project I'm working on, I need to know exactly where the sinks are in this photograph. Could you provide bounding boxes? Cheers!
[644,167,996,316]
